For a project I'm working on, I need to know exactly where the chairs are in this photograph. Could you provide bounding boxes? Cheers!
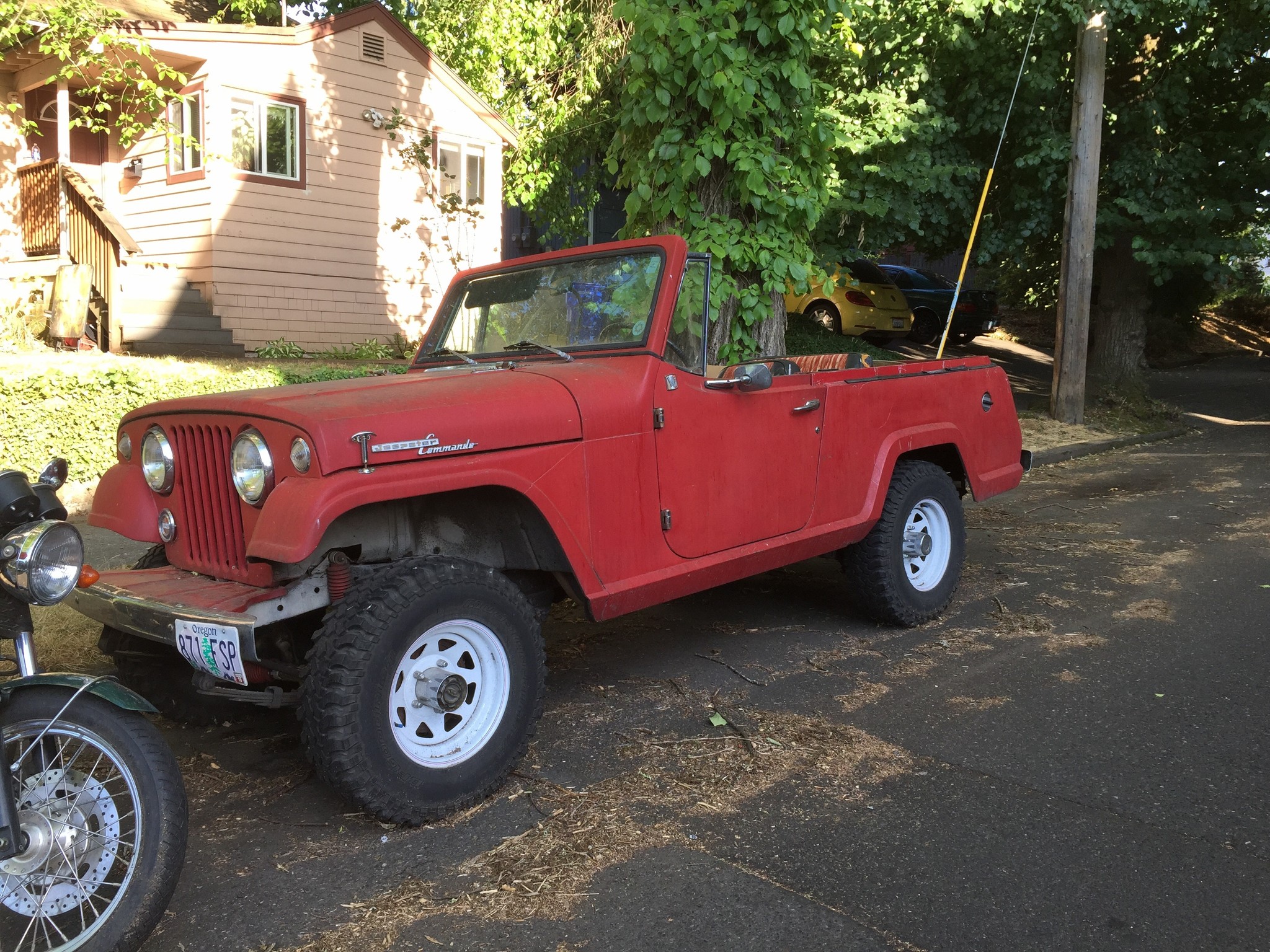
[716,359,800,379]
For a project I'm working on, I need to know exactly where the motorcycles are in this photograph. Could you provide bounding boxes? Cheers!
[0,455,188,952]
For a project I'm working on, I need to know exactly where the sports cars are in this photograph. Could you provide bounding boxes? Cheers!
[56,231,1037,829]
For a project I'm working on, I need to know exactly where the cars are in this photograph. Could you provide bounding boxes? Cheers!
[782,252,915,346]
[877,264,999,347]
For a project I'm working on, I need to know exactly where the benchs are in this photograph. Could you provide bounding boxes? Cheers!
[741,352,873,375]
